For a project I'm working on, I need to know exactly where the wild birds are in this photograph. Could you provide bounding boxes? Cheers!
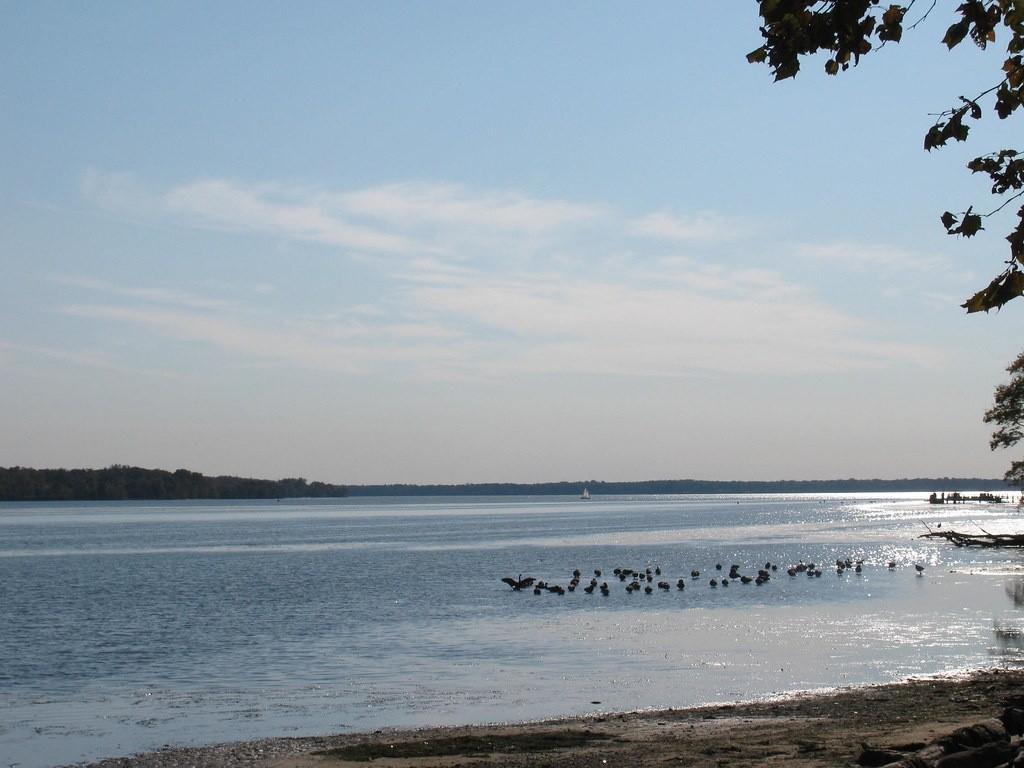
[856,558,865,564]
[888,562,896,570]
[938,523,941,528]
[915,564,924,574]
[496,560,823,594]
[835,557,852,575]
[855,565,862,574]
